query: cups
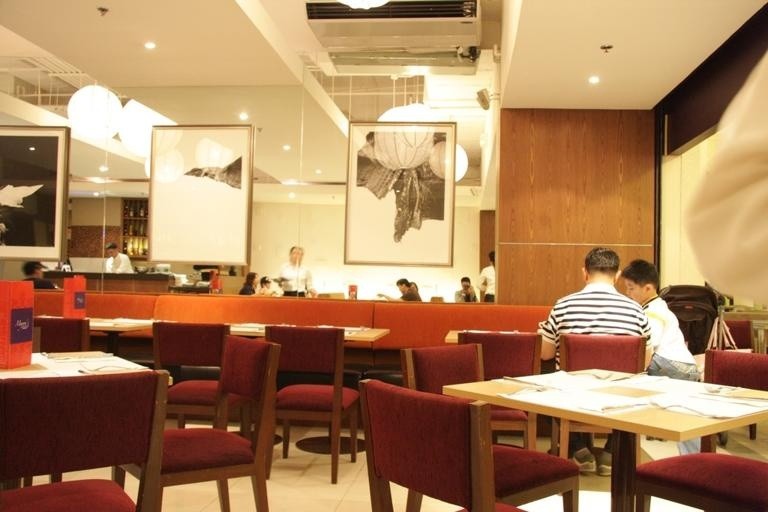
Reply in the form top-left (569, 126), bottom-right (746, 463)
top-left (349, 285), bottom-right (357, 300)
top-left (753, 297), bottom-right (763, 311)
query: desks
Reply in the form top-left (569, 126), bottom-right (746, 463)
top-left (445, 330), bottom-right (537, 345)
top-left (171, 286), bottom-right (210, 294)
top-left (442, 367), bottom-right (768, 512)
top-left (0, 351), bottom-right (174, 491)
top-left (227, 322), bottom-right (390, 342)
top-left (35, 315), bottom-right (176, 331)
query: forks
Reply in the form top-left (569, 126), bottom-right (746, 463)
top-left (717, 385), bottom-right (742, 391)
top-left (657, 403), bottom-right (705, 417)
top-left (85, 364), bottom-right (130, 372)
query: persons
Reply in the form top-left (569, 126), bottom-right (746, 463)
top-left (536, 246), bottom-right (655, 477)
top-left (454, 276), bottom-right (478, 302)
top-left (621, 259), bottom-right (702, 456)
top-left (21, 261), bottom-right (55, 289)
top-left (239, 272), bottom-right (259, 295)
top-left (408, 282), bottom-right (418, 291)
top-left (384, 278), bottom-right (423, 302)
top-left (259, 276), bottom-right (272, 296)
top-left (105, 242), bottom-right (134, 273)
top-left (272, 246), bottom-right (316, 297)
top-left (476, 250), bottom-right (496, 303)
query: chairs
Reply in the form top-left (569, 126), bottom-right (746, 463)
top-left (0, 369), bottom-right (169, 512)
top-left (633, 453), bottom-right (768, 511)
top-left (152, 322), bottom-right (252, 443)
top-left (33, 316), bottom-right (90, 353)
top-left (403, 343), bottom-right (526, 512)
top-left (457, 332), bottom-right (542, 451)
top-left (706, 350), bottom-right (768, 390)
top-left (266, 326), bottom-right (361, 484)
top-left (363, 378), bottom-right (579, 512)
top-left (110, 335), bottom-right (280, 512)
top-left (549, 334), bottom-right (650, 460)
top-left (724, 320), bottom-right (753, 351)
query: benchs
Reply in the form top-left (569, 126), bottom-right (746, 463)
top-left (352, 305), bottom-right (554, 385)
top-left (151, 295), bottom-right (374, 390)
top-left (32, 292), bottom-right (156, 367)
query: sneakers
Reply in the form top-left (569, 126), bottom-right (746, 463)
top-left (598, 464), bottom-right (612, 476)
top-left (571, 453), bottom-right (596, 473)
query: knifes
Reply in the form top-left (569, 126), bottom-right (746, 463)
top-left (602, 404), bottom-right (649, 412)
top-left (612, 372), bottom-right (648, 381)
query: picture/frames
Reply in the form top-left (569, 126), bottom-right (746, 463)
top-left (0, 125), bottom-right (70, 261)
top-left (347, 120), bottom-right (456, 268)
top-left (146, 124), bottom-right (253, 268)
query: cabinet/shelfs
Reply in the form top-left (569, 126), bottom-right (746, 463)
top-left (42, 271), bottom-right (176, 294)
top-left (123, 199), bottom-right (147, 258)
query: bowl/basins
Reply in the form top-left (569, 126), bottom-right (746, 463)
top-left (135, 266), bottom-right (149, 273)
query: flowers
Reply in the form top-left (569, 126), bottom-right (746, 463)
top-left (355, 129), bottom-right (447, 241)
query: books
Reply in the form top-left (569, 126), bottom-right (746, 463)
top-left (0, 279), bottom-right (35, 370)
top-left (62, 277), bottom-right (86, 319)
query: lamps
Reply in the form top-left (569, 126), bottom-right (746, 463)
top-left (396, 104), bottom-right (430, 127)
top-left (146, 41), bottom-right (154, 49)
top-left (67, 86), bottom-right (123, 141)
top-left (145, 149), bottom-right (152, 177)
top-left (454, 145), bottom-right (468, 183)
top-left (589, 75), bottom-right (596, 83)
top-left (119, 99), bottom-right (180, 156)
top-left (239, 112), bottom-right (247, 120)
top-left (339, 0), bottom-right (387, 9)
top-left (377, 105), bottom-right (397, 124)
